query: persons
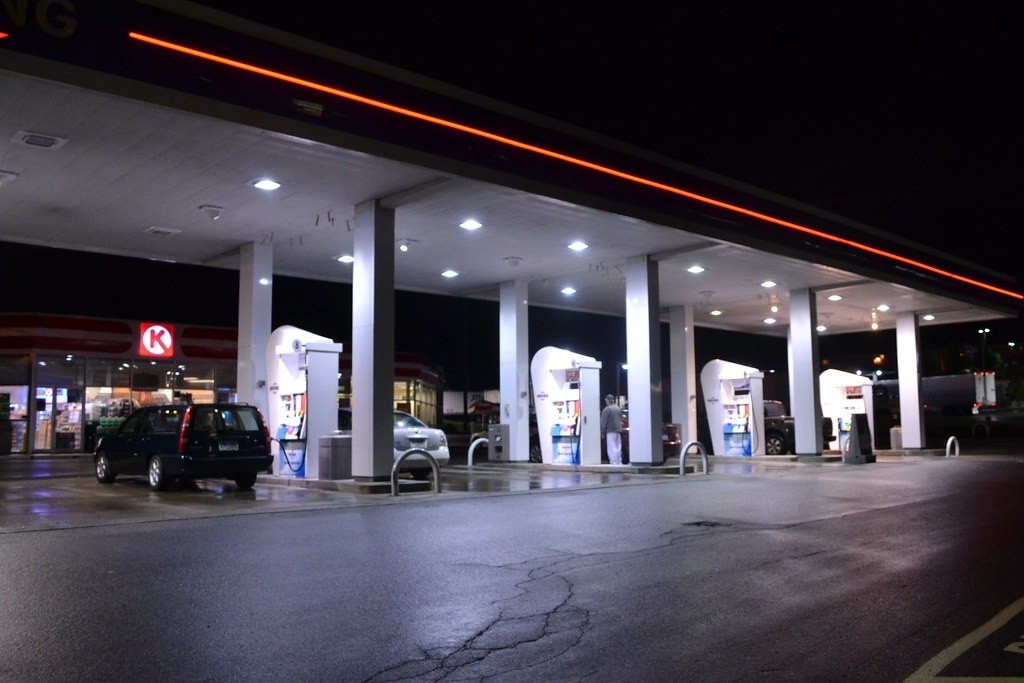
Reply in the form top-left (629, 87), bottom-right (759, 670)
top-left (601, 394), bottom-right (624, 466)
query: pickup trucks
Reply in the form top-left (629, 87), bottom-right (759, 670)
top-left (762, 400), bottom-right (837, 455)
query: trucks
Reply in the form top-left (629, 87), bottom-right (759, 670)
top-left (872, 371), bottom-right (1002, 449)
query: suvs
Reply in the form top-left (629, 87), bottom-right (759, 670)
top-left (94, 403), bottom-right (274, 490)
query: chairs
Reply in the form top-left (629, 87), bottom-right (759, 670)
top-left (150, 414), bottom-right (237, 432)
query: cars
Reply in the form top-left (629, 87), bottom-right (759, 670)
top-left (338, 407), bottom-right (449, 479)
top-left (601, 408), bottom-right (683, 465)
